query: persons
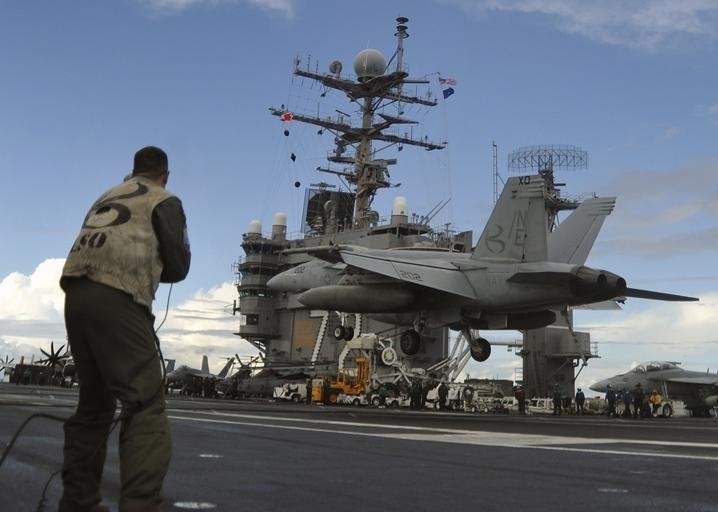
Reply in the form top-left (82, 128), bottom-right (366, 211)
top-left (462, 382), bottom-right (474, 405)
top-left (604, 382), bottom-right (661, 418)
top-left (306, 376), bottom-right (330, 405)
top-left (365, 380), bottom-right (387, 408)
top-left (515, 386), bottom-right (525, 412)
top-left (552, 384), bottom-right (561, 414)
top-left (575, 388), bottom-right (585, 416)
top-left (57, 146), bottom-right (192, 512)
top-left (410, 378), bottom-right (448, 411)
top-left (192, 376), bottom-right (216, 398)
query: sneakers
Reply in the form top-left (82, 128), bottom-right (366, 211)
top-left (159, 498), bottom-right (175, 510)
top-left (60, 502), bottom-right (109, 511)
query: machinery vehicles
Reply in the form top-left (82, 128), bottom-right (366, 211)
top-left (329, 358), bottom-right (370, 403)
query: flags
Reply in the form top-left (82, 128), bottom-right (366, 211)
top-left (438, 79), bottom-right (458, 86)
top-left (281, 112), bottom-right (293, 122)
top-left (442, 87), bottom-right (454, 98)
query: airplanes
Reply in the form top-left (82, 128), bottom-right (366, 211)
top-left (589, 363), bottom-right (718, 418)
top-left (266, 175), bottom-right (699, 362)
top-left (1, 344), bottom-right (258, 399)
